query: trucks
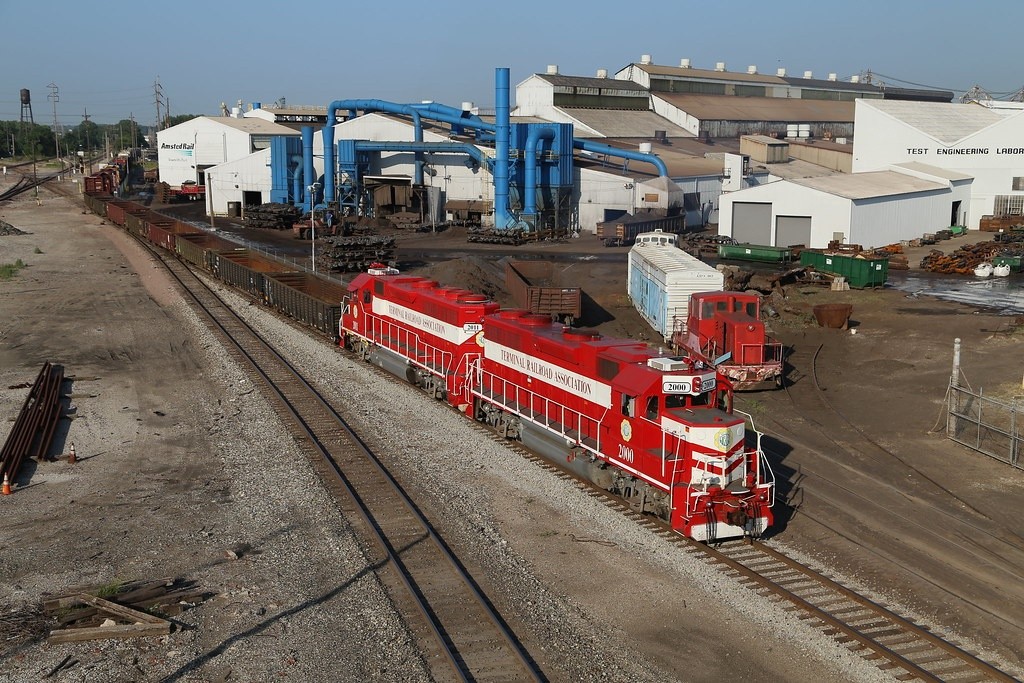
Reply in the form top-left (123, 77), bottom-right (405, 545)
top-left (627, 240), bottom-right (784, 394)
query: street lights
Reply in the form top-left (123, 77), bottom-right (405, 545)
top-left (306, 182), bottom-right (321, 273)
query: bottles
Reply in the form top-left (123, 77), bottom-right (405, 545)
top-left (70, 443), bottom-right (76, 462)
top-left (3, 472), bottom-right (10, 495)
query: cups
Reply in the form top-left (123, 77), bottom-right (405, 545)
top-left (851, 329), bottom-right (856, 334)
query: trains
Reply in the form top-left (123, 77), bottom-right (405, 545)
top-left (83, 147), bottom-right (777, 543)
